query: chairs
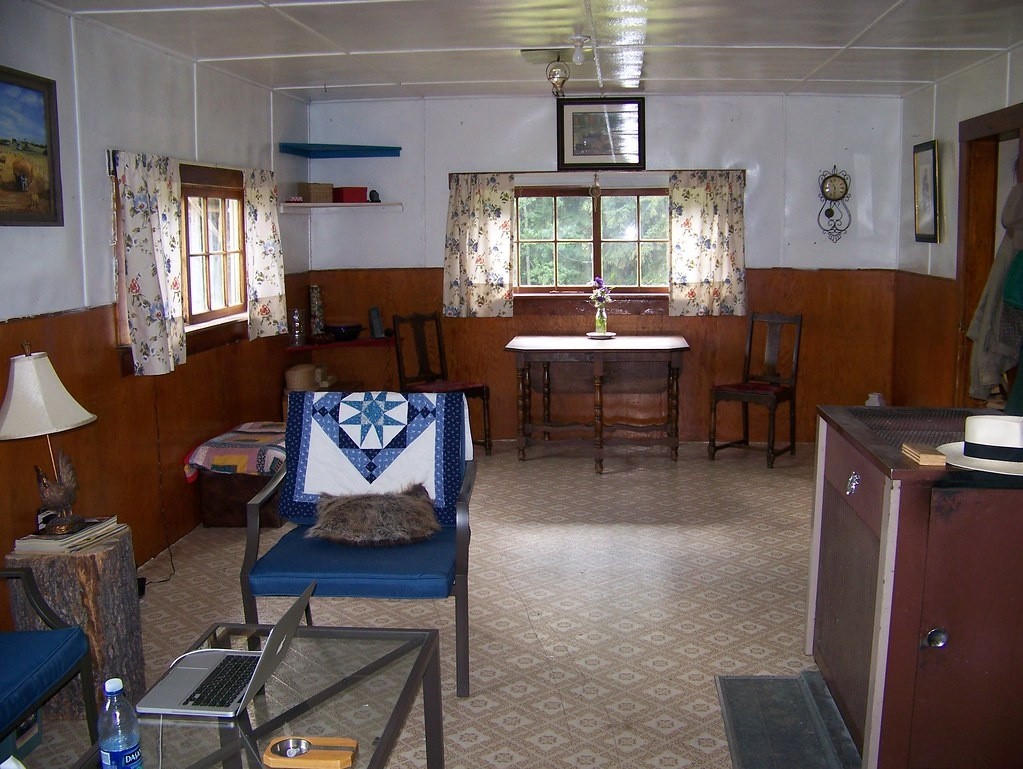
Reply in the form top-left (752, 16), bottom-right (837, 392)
top-left (393, 310), bottom-right (493, 456)
top-left (709, 311), bottom-right (804, 470)
top-left (239, 390), bottom-right (477, 699)
top-left (0, 567), bottom-right (99, 747)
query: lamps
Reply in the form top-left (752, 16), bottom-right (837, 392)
top-left (0, 339), bottom-right (98, 535)
top-left (545, 56), bottom-right (570, 97)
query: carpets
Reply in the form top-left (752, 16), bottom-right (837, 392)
top-left (713, 669), bottom-right (863, 769)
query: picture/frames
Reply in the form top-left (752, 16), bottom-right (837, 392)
top-left (368, 307), bottom-right (385, 339)
top-left (0, 64), bottom-right (64, 227)
top-left (913, 139), bottom-right (940, 244)
top-left (556, 98), bottom-right (646, 172)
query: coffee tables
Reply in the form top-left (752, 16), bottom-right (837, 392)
top-left (70, 622), bottom-right (445, 769)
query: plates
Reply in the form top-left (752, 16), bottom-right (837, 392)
top-left (586, 332), bottom-right (617, 338)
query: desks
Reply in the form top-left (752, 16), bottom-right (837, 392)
top-left (6, 523), bottom-right (147, 721)
top-left (504, 335), bottom-right (691, 475)
top-left (281, 381), bottom-right (364, 422)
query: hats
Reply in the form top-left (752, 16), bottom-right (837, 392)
top-left (935, 414), bottom-right (1023, 477)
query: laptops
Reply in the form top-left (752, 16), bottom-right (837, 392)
top-left (135, 580), bottom-right (318, 718)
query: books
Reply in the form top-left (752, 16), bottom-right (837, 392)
top-left (14, 514), bottom-right (127, 553)
top-left (902, 443), bottom-right (947, 466)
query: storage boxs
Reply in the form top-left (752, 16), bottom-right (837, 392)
top-left (333, 187), bottom-right (367, 202)
top-left (297, 182), bottom-right (333, 203)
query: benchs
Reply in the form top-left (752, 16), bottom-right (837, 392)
top-left (184, 422), bottom-right (288, 527)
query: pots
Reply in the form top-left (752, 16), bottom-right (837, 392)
top-left (320, 323), bottom-right (367, 340)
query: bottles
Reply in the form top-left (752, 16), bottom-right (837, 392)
top-left (96, 678), bottom-right (144, 769)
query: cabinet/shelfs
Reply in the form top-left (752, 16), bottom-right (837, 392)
top-left (801, 405), bottom-right (1023, 769)
top-left (278, 142), bottom-right (403, 360)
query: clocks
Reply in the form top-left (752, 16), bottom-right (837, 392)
top-left (817, 164), bottom-right (852, 244)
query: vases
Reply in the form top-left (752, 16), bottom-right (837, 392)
top-left (595, 308), bottom-right (607, 335)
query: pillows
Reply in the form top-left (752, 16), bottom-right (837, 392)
top-left (305, 482), bottom-right (442, 549)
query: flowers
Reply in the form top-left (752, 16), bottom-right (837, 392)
top-left (584, 276), bottom-right (617, 331)
